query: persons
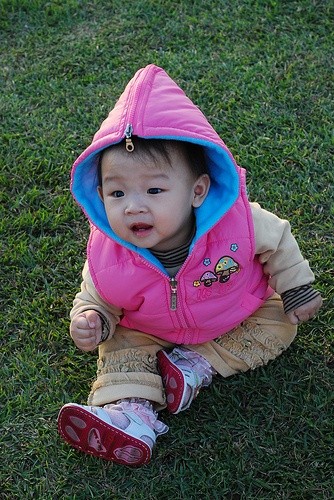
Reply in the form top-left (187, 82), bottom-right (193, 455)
top-left (55, 63), bottom-right (323, 468)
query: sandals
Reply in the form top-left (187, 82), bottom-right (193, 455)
top-left (156, 347), bottom-right (203, 414)
top-left (57, 401), bottom-right (157, 467)
